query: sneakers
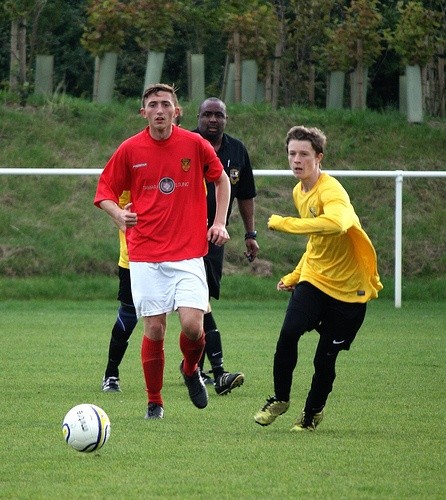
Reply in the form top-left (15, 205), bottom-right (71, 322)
top-left (213, 371), bottom-right (245, 396)
top-left (145, 401), bottom-right (165, 420)
top-left (253, 394), bottom-right (290, 426)
top-left (200, 370), bottom-right (214, 384)
top-left (101, 373), bottom-right (122, 392)
top-left (179, 359), bottom-right (209, 409)
top-left (289, 408), bottom-right (324, 435)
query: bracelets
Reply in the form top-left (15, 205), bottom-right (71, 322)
top-left (244, 231), bottom-right (257, 237)
top-left (245, 236), bottom-right (256, 241)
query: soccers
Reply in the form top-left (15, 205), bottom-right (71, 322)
top-left (62, 403), bottom-right (111, 453)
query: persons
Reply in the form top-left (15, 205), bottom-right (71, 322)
top-left (101, 97), bottom-right (260, 396)
top-left (253, 123), bottom-right (384, 433)
top-left (93, 83), bottom-right (232, 420)
